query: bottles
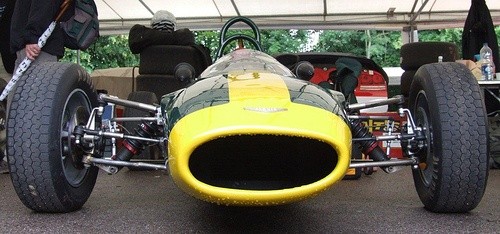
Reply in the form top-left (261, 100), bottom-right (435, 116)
top-left (437, 56), bottom-right (443, 63)
top-left (480, 43), bottom-right (493, 81)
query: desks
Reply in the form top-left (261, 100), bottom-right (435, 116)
top-left (477, 80), bottom-right (500, 101)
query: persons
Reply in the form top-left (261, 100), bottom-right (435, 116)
top-left (0, 0), bottom-right (74, 174)
top-left (151, 10), bottom-right (176, 32)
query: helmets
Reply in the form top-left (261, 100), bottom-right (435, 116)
top-left (151, 10), bottom-right (176, 32)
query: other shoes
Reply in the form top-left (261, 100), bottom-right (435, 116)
top-left (0, 161), bottom-right (10, 173)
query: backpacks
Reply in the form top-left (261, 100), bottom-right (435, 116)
top-left (60, 0), bottom-right (101, 50)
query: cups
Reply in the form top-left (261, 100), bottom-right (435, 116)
top-left (495, 73), bottom-right (500, 80)
